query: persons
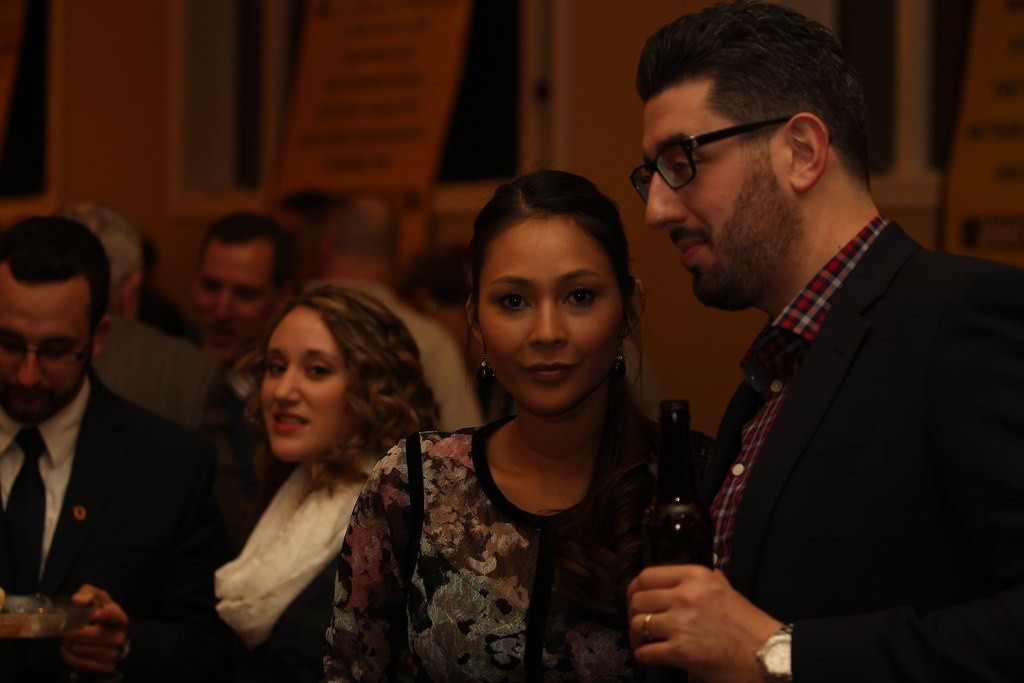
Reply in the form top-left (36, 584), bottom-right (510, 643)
top-left (626, 0), bottom-right (1024, 683)
top-left (216, 285), bottom-right (443, 683)
top-left (64, 200), bottom-right (243, 496)
top-left (304, 196), bottom-right (483, 433)
top-left (323, 170), bottom-right (714, 683)
top-left (198, 213), bottom-right (300, 502)
top-left (0, 212), bottom-right (233, 683)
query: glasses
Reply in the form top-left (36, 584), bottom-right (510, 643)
top-left (631, 113), bottom-right (839, 205)
top-left (0, 329), bottom-right (94, 369)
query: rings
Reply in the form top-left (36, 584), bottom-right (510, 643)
top-left (642, 615), bottom-right (654, 641)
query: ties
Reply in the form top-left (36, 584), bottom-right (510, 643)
top-left (4, 427), bottom-right (45, 594)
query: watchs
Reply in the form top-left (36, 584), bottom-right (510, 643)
top-left (756, 624), bottom-right (794, 683)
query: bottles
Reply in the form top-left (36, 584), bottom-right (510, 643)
top-left (647, 400), bottom-right (712, 568)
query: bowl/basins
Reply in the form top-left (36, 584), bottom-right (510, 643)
top-left (0, 595), bottom-right (98, 638)
